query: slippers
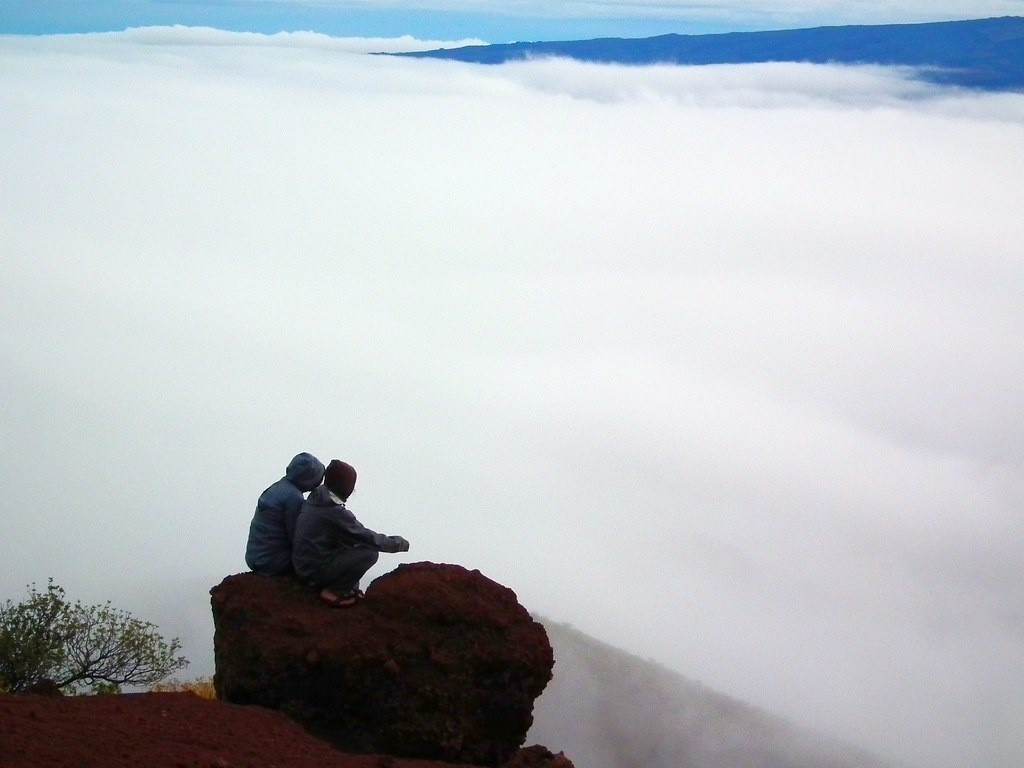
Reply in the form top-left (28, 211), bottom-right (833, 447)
top-left (327, 591), bottom-right (363, 608)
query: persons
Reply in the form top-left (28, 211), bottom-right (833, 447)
top-left (292, 459), bottom-right (409, 606)
top-left (245, 452), bottom-right (325, 575)
